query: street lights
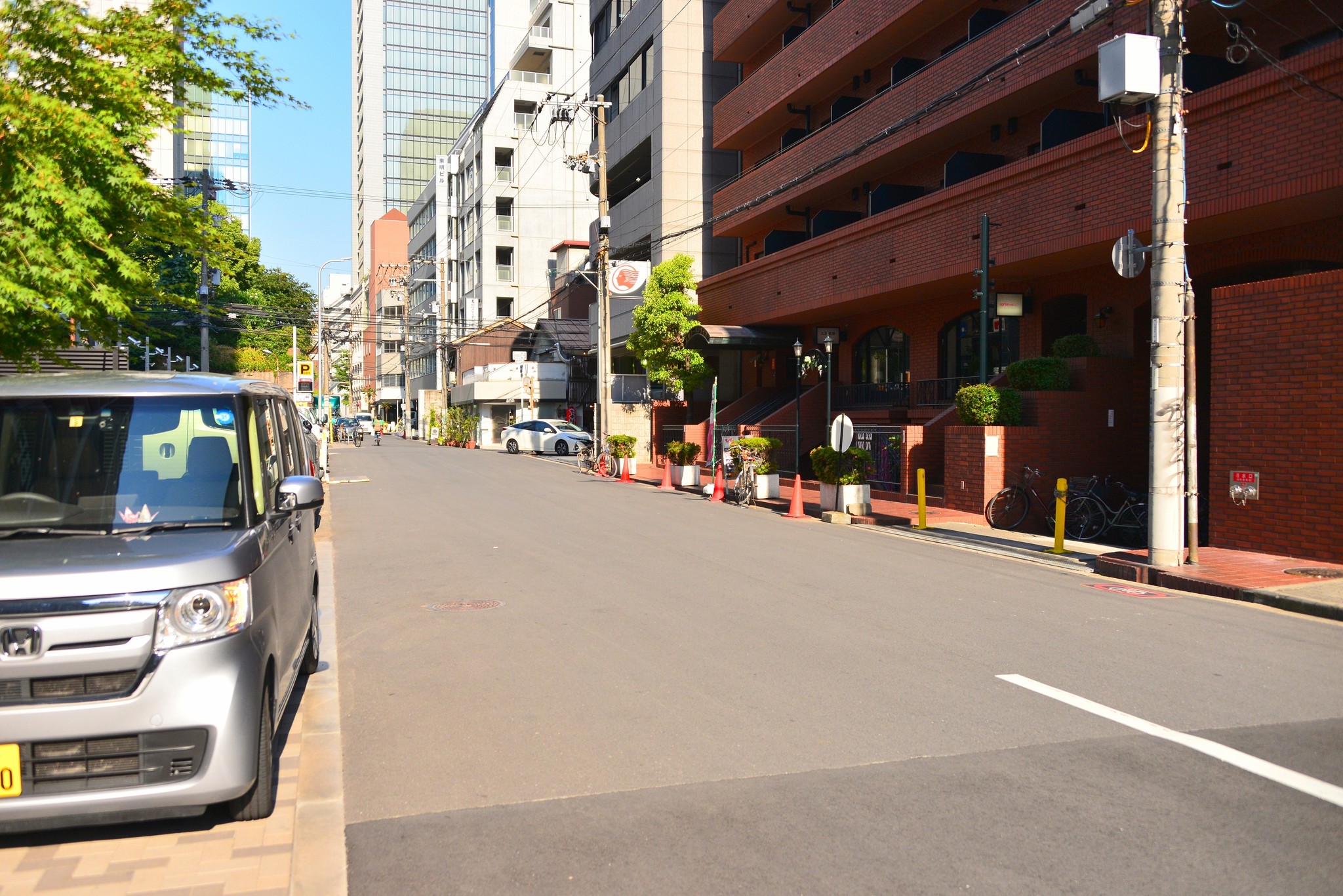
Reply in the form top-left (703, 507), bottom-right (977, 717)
top-left (317, 256), bottom-right (355, 427)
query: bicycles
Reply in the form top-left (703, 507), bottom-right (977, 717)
top-left (1064, 473), bottom-right (1150, 545)
top-left (724, 444), bottom-right (765, 508)
top-left (988, 463), bottom-right (1095, 541)
top-left (396, 421), bottom-right (403, 433)
top-left (350, 423), bottom-right (364, 448)
top-left (577, 432), bottom-right (618, 478)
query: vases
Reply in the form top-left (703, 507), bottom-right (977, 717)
top-left (612, 457), bottom-right (636, 475)
top-left (739, 473), bottom-right (781, 499)
top-left (820, 482), bottom-right (872, 515)
top-left (670, 464), bottom-right (700, 486)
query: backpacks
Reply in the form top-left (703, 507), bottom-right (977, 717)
top-left (374, 420), bottom-right (382, 431)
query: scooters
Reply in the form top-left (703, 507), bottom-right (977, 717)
top-left (372, 425), bottom-right (382, 446)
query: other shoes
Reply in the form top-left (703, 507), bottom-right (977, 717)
top-left (374, 439), bottom-right (377, 442)
top-left (379, 438), bottom-right (382, 439)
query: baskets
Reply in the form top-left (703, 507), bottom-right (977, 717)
top-left (601, 441), bottom-right (614, 453)
top-left (739, 452), bottom-right (763, 469)
top-left (1006, 468), bottom-right (1034, 489)
top-left (1068, 476), bottom-right (1091, 495)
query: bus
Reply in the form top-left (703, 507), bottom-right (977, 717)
top-left (402, 412), bottom-right (418, 429)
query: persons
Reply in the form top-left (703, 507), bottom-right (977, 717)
top-left (372, 415), bottom-right (384, 443)
top-left (331, 407), bottom-right (340, 418)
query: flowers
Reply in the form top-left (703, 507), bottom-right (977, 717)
top-left (666, 440), bottom-right (702, 466)
top-left (729, 436), bottom-right (784, 475)
top-left (605, 434), bottom-right (638, 459)
top-left (810, 445), bottom-right (877, 485)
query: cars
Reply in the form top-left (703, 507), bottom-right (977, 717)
top-left (295, 406), bottom-right (327, 482)
top-left (334, 418), bottom-right (364, 441)
top-left (501, 419), bottom-right (595, 454)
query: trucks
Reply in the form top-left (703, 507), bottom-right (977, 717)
top-left (313, 395), bottom-right (342, 424)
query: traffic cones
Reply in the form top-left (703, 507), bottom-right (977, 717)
top-left (706, 465), bottom-right (727, 503)
top-left (780, 474), bottom-right (812, 520)
top-left (657, 458), bottom-right (675, 490)
top-left (615, 454), bottom-right (635, 483)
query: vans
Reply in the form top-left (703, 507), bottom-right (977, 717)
top-left (0, 369), bottom-right (329, 834)
top-left (354, 413), bottom-right (373, 434)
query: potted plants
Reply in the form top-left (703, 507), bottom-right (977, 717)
top-left (437, 401), bottom-right (480, 449)
